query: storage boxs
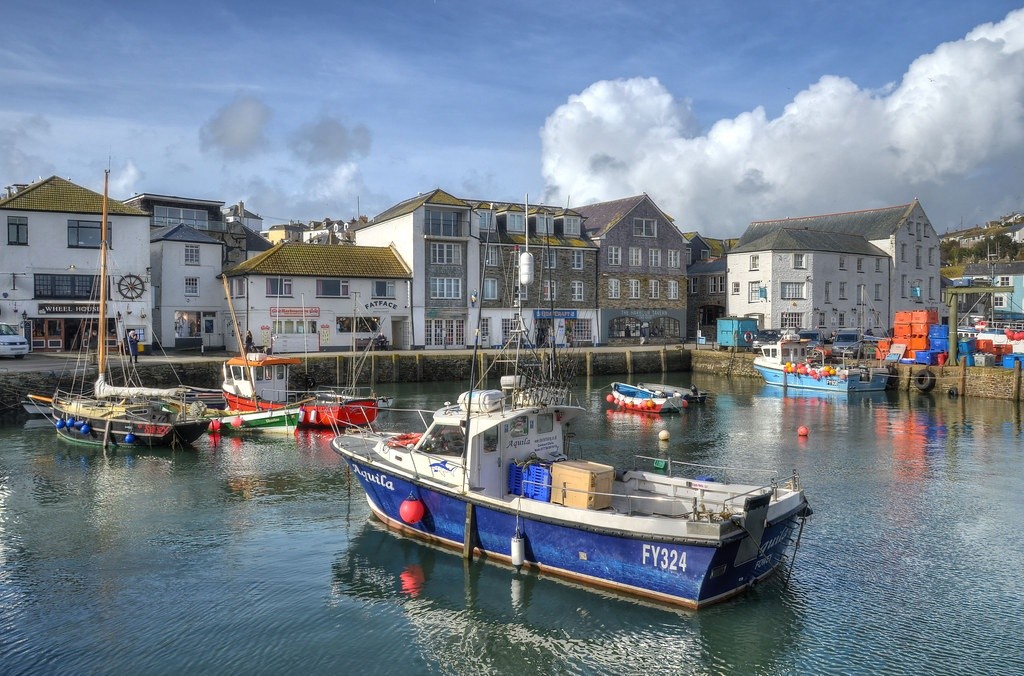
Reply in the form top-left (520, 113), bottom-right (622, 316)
top-left (506, 463), bottom-right (554, 501)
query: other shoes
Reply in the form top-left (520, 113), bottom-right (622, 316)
top-left (135, 362), bottom-right (139, 363)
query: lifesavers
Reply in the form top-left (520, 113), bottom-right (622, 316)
top-left (948, 387), bottom-right (958, 394)
top-left (913, 368), bottom-right (936, 391)
top-left (385, 432), bottom-right (422, 448)
top-left (743, 331), bottom-right (753, 342)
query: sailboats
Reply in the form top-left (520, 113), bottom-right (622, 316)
top-left (2, 156), bottom-right (315, 449)
top-left (221, 274), bottom-right (394, 429)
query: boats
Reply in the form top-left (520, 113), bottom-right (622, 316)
top-left (323, 193), bottom-right (814, 610)
top-left (753, 283), bottom-right (891, 393)
top-left (604, 382), bottom-right (712, 414)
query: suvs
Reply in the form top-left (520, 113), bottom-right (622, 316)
top-left (752, 329), bottom-right (784, 354)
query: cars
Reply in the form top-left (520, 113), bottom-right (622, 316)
top-left (0, 322), bottom-right (29, 358)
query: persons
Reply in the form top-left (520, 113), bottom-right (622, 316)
top-left (245, 330), bottom-right (252, 353)
top-left (129, 330), bottom-right (140, 364)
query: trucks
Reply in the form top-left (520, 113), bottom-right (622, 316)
top-left (831, 328), bottom-right (865, 358)
